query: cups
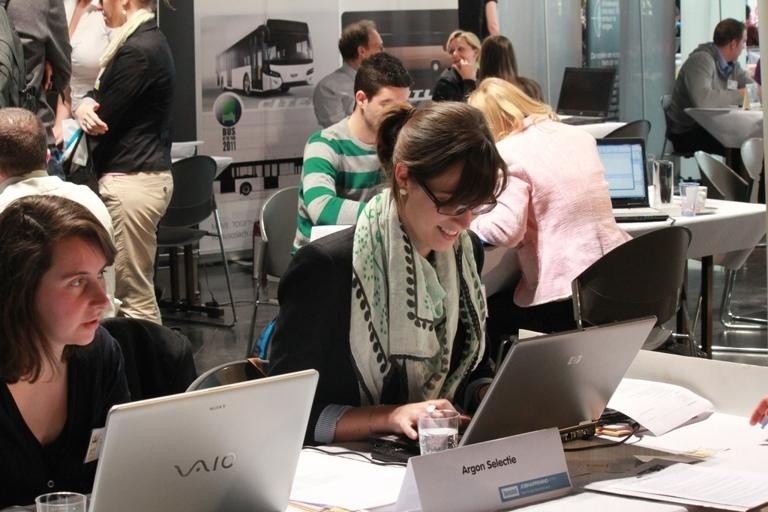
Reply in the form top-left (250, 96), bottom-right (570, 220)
top-left (679, 182), bottom-right (700, 217)
top-left (417, 410), bottom-right (459, 456)
top-left (35, 492), bottom-right (87, 512)
top-left (686, 186), bottom-right (708, 213)
top-left (653, 160), bottom-right (675, 204)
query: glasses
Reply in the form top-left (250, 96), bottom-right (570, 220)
top-left (417, 178), bottom-right (497, 216)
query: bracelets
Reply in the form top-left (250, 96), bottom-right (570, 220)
top-left (368, 404), bottom-right (387, 435)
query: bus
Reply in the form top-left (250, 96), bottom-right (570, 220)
top-left (213, 18), bottom-right (316, 98)
top-left (375, 28), bottom-right (457, 74)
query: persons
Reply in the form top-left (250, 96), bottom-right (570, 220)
top-left (429, 28), bottom-right (483, 102)
top-left (0, 193), bottom-right (133, 510)
top-left (667, 17), bottom-right (761, 156)
top-left (288, 51), bottom-right (416, 259)
top-left (746, 4), bottom-right (758, 47)
top-left (458, 0), bottom-right (499, 43)
top-left (312, 17), bottom-right (386, 128)
top-left (463, 71), bottom-right (633, 358)
top-left (477, 34), bottom-right (543, 104)
top-left (267, 98), bottom-right (511, 445)
top-left (1, 1), bottom-right (177, 327)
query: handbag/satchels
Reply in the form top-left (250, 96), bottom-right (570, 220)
top-left (62, 128), bottom-right (99, 195)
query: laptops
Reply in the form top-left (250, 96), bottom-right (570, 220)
top-left (596, 137), bottom-right (669, 223)
top-left (557, 68), bottom-right (616, 118)
top-left (369, 315), bottom-right (658, 462)
top-left (0, 369), bottom-right (320, 512)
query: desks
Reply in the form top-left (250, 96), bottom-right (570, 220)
top-left (0, 417), bottom-right (766, 511)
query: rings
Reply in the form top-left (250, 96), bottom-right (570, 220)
top-left (424, 404), bottom-right (437, 415)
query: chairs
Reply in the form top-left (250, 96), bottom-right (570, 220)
top-left (244, 187), bottom-right (299, 357)
top-left (151, 139), bottom-right (237, 331)
